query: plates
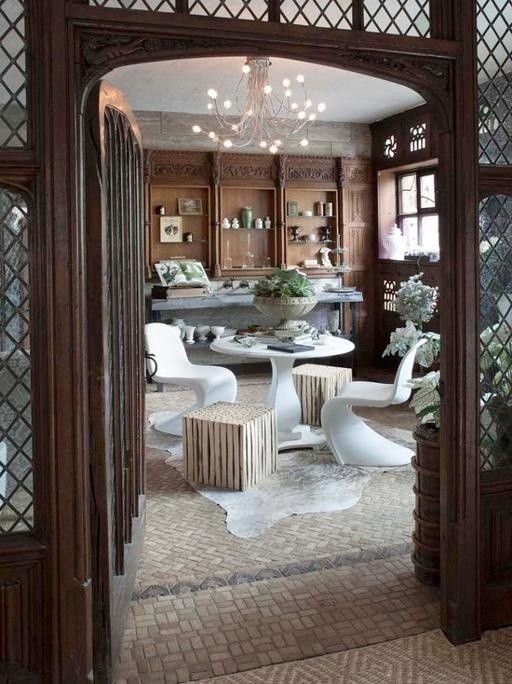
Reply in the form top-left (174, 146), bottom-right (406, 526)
top-left (238, 329), bottom-right (270, 335)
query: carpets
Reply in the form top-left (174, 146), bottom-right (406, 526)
top-left (147, 413), bottom-right (412, 539)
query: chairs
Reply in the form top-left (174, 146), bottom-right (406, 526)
top-left (320, 338), bottom-right (429, 466)
top-left (144, 322), bottom-right (238, 437)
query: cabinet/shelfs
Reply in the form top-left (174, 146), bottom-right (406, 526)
top-left (143, 151), bottom-right (341, 281)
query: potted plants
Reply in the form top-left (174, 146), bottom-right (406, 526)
top-left (381, 271), bottom-right (512, 586)
top-left (251, 268), bottom-right (318, 341)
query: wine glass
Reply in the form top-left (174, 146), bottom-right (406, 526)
top-left (210, 326), bottom-right (226, 341)
top-left (183, 326), bottom-right (196, 343)
top-left (195, 324), bottom-right (210, 341)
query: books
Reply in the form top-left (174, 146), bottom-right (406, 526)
top-left (267, 341), bottom-right (316, 353)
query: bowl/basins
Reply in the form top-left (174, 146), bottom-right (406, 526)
top-left (248, 325), bottom-right (263, 332)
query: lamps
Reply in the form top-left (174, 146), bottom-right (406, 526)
top-left (191, 56), bottom-right (328, 154)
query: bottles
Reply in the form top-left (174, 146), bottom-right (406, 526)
top-left (223, 240), bottom-right (233, 270)
top-left (259, 237), bottom-right (272, 269)
top-left (241, 230), bottom-right (256, 269)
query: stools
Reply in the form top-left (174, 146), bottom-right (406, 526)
top-left (181, 401), bottom-right (277, 491)
top-left (292, 363), bottom-right (353, 427)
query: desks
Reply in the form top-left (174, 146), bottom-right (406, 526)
top-left (209, 334), bottom-right (355, 452)
